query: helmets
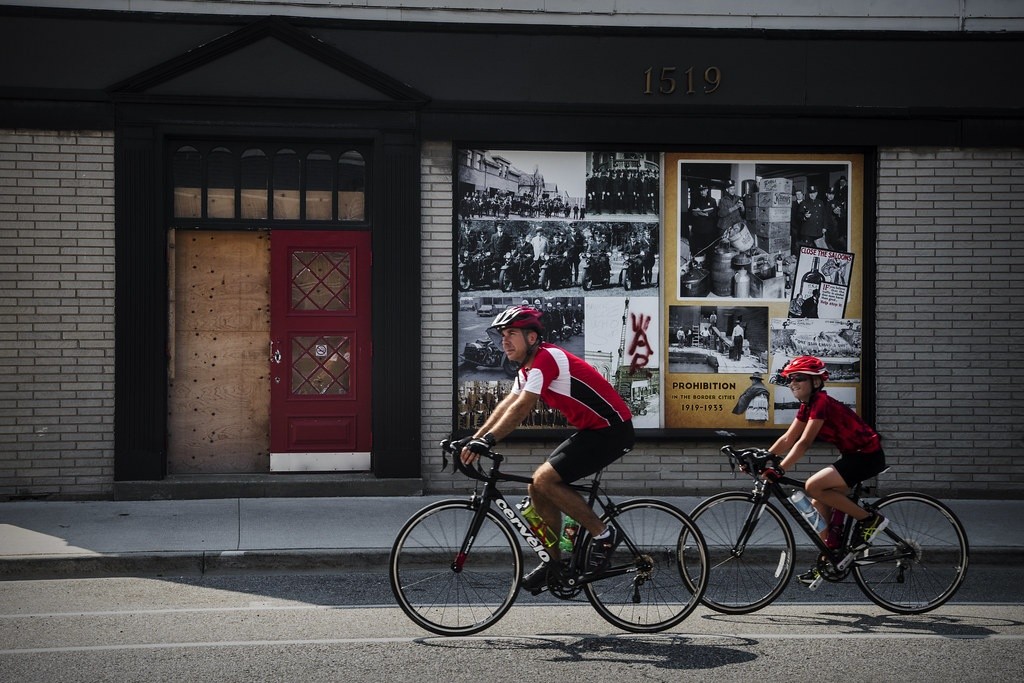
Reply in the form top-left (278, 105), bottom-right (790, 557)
top-left (780, 356), bottom-right (829, 381)
top-left (485, 306), bottom-right (546, 335)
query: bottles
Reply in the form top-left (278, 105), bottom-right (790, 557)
top-left (559, 514), bottom-right (579, 553)
top-left (790, 488), bottom-right (827, 533)
top-left (515, 496), bottom-right (558, 548)
top-left (777, 251), bottom-right (783, 272)
top-left (761, 261), bottom-right (775, 278)
top-left (825, 507), bottom-right (845, 548)
top-left (784, 276), bottom-right (792, 299)
top-left (800, 256), bottom-right (825, 301)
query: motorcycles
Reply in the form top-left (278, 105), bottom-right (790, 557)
top-left (459, 322), bottom-right (582, 378)
top-left (540, 255), bottom-right (573, 291)
top-left (457, 250), bottom-right (541, 292)
top-left (579, 253), bottom-right (610, 291)
top-left (622, 251), bottom-right (645, 290)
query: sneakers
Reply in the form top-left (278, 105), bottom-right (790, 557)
top-left (849, 509), bottom-right (890, 550)
top-left (796, 565), bottom-right (820, 584)
top-left (521, 561), bottom-right (551, 591)
top-left (581, 524), bottom-right (626, 580)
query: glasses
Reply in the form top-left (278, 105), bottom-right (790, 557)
top-left (786, 376), bottom-right (807, 382)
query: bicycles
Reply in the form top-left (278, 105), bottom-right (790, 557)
top-left (677, 445), bottom-right (969, 615)
top-left (389, 436), bottom-right (710, 636)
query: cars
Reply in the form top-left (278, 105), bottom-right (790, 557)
top-left (477, 305), bottom-right (498, 317)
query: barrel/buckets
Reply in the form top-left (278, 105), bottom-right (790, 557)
top-left (723, 222), bottom-right (754, 252)
top-left (708, 248), bottom-right (739, 297)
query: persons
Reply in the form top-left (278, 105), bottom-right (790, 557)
top-left (744, 356), bottom-right (888, 587)
top-left (458, 167), bottom-right (859, 427)
top-left (454, 305), bottom-right (635, 589)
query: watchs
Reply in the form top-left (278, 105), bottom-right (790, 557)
top-left (482, 432), bottom-right (498, 447)
